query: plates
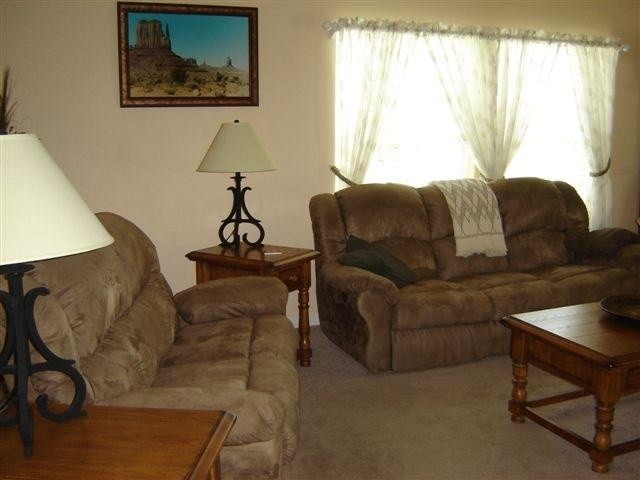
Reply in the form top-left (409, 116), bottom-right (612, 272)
top-left (600, 292), bottom-right (640, 321)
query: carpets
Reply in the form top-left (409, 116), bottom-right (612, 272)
top-left (294, 325), bottom-right (640, 479)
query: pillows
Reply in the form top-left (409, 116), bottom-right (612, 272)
top-left (336, 234), bottom-right (419, 289)
top-left (570, 227), bottom-right (636, 261)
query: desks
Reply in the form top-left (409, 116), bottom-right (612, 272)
top-left (0, 401), bottom-right (237, 478)
top-left (185, 241), bottom-right (322, 367)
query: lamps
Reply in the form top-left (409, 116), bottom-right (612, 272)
top-left (196, 119), bottom-right (282, 251)
top-left (0, 134), bottom-right (114, 458)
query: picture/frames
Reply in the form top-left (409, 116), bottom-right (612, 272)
top-left (116, 2), bottom-right (259, 108)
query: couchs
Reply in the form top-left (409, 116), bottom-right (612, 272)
top-left (309, 178), bottom-right (639, 375)
top-left (0, 211), bottom-right (301, 480)
